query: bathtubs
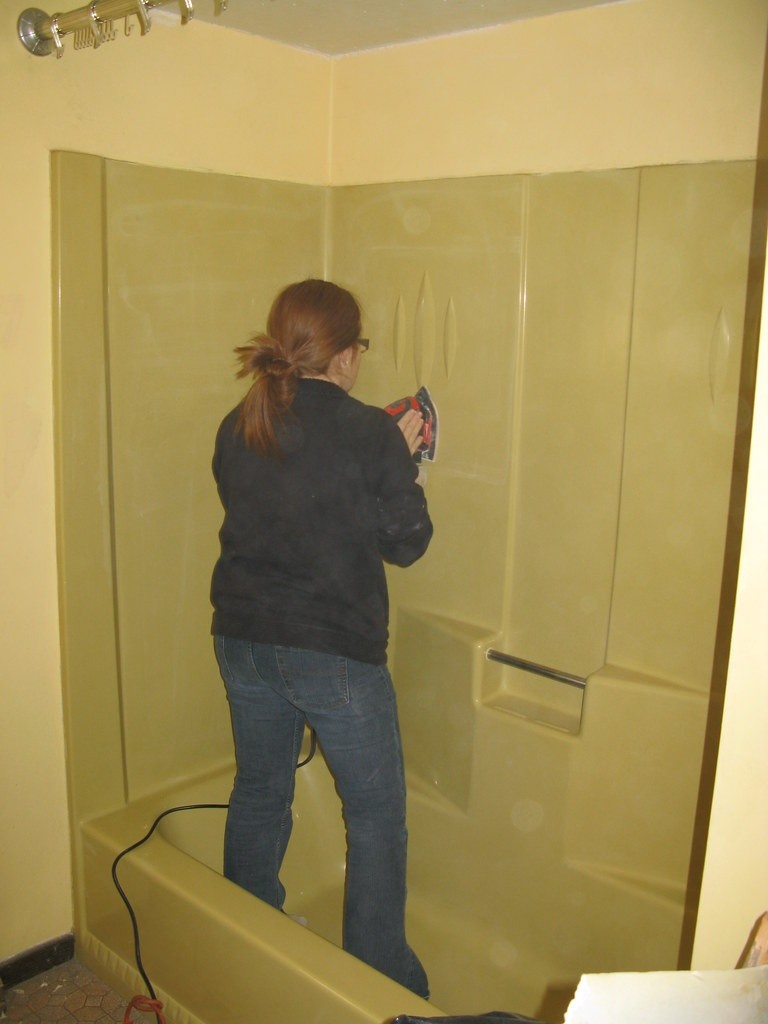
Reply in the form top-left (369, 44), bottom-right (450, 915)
top-left (77, 736), bottom-right (692, 1024)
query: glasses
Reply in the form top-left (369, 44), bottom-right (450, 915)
top-left (355, 339), bottom-right (369, 353)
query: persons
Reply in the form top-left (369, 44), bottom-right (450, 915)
top-left (209, 280), bottom-right (433, 1002)
